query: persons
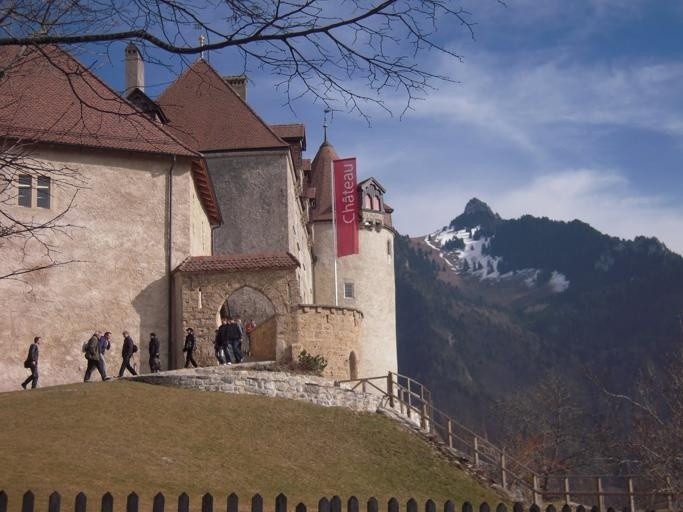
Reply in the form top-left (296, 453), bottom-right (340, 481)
top-left (182, 328), bottom-right (198, 368)
top-left (21, 337), bottom-right (41, 390)
top-left (91, 331), bottom-right (112, 377)
top-left (84, 331), bottom-right (111, 382)
top-left (214, 315), bottom-right (256, 367)
top-left (148, 332), bottom-right (160, 373)
top-left (113, 331), bottom-right (137, 377)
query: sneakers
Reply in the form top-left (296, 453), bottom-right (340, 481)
top-left (103, 377), bottom-right (110, 381)
top-left (84, 380), bottom-right (91, 382)
top-left (22, 383), bottom-right (25, 389)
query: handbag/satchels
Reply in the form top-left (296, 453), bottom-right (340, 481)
top-left (82, 340), bottom-right (89, 352)
top-left (152, 357), bottom-right (160, 369)
top-left (24, 360), bottom-right (29, 367)
top-left (133, 345), bottom-right (137, 352)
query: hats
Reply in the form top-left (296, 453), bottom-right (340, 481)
top-left (186, 328), bottom-right (193, 332)
